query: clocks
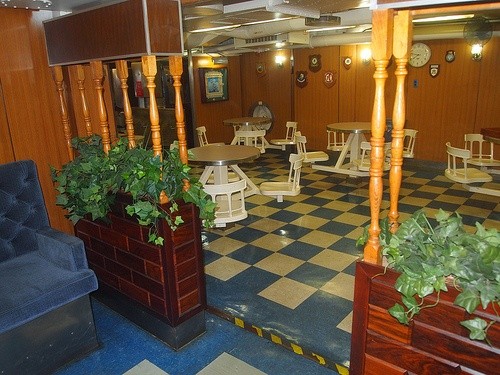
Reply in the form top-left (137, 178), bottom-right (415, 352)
top-left (408, 43), bottom-right (431, 67)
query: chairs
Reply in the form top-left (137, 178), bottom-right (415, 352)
top-left (271, 121), bottom-right (297, 150)
top-left (462, 133), bottom-right (500, 168)
top-left (352, 142), bottom-right (391, 171)
top-left (259, 153), bottom-right (304, 203)
top-left (195, 125), bottom-right (224, 147)
top-left (202, 179), bottom-right (248, 227)
top-left (294, 131), bottom-right (329, 163)
top-left (403, 129), bottom-right (419, 158)
top-left (0, 160), bottom-right (104, 375)
top-left (327, 130), bottom-right (347, 152)
top-left (235, 130), bottom-right (266, 153)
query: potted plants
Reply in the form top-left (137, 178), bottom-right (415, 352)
top-left (355, 208), bottom-right (500, 348)
top-left (48, 130), bottom-right (219, 246)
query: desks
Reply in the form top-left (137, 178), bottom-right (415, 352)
top-left (480, 128), bottom-right (500, 145)
top-left (326, 122), bottom-right (387, 178)
top-left (188, 145), bottom-right (262, 198)
top-left (223, 117), bottom-right (272, 152)
top-left (444, 142), bottom-right (493, 186)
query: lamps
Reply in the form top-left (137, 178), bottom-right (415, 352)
top-left (305, 16), bottom-right (341, 26)
